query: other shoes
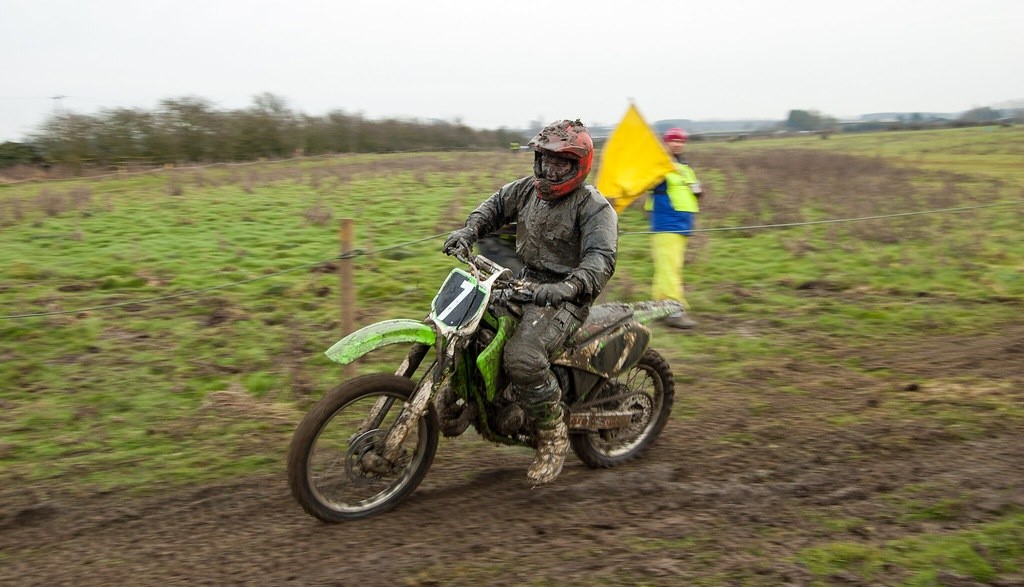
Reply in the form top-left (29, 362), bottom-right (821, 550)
top-left (663, 317), bottom-right (697, 329)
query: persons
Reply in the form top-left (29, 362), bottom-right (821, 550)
top-left (442, 120), bottom-right (618, 485)
top-left (645, 127), bottom-right (705, 327)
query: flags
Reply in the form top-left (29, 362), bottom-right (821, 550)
top-left (593, 105), bottom-right (677, 216)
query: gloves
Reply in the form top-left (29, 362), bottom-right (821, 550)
top-left (532, 280), bottom-right (578, 311)
top-left (441, 221), bottom-right (480, 256)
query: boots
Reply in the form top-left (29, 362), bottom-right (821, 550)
top-left (527, 410), bottom-right (571, 483)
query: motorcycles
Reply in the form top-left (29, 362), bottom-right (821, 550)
top-left (288, 241), bottom-right (676, 523)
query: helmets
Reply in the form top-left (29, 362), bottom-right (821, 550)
top-left (663, 127), bottom-right (688, 142)
top-left (527, 120), bottom-right (594, 201)
